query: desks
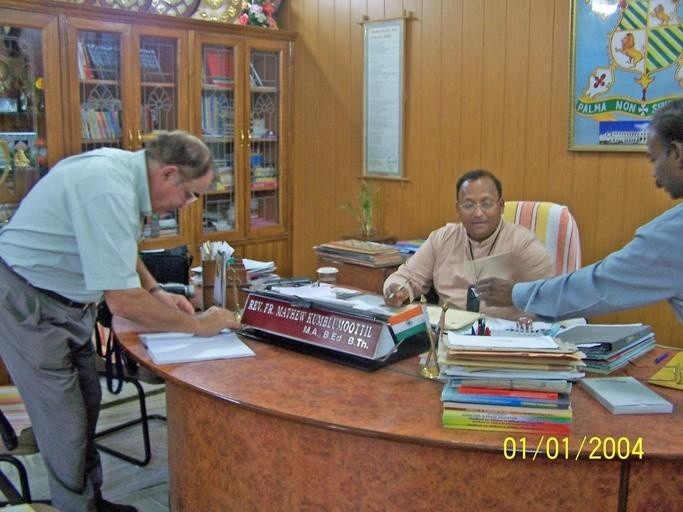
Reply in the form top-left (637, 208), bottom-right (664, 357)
top-left (343, 233), bottom-right (400, 247)
top-left (112, 269), bottom-right (682, 512)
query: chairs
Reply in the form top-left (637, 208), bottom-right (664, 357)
top-left (1, 396), bottom-right (100, 512)
top-left (499, 198), bottom-right (589, 331)
top-left (91, 244), bottom-right (199, 468)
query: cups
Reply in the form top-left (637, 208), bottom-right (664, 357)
top-left (315, 266), bottom-right (340, 286)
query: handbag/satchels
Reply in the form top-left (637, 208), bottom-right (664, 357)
top-left (97, 245), bottom-right (195, 330)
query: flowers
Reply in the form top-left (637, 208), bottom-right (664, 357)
top-left (238, 1), bottom-right (280, 29)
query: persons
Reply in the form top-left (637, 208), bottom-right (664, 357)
top-left (471, 94), bottom-right (683, 323)
top-left (381, 168), bottom-right (556, 323)
top-left (0, 127), bottom-right (242, 511)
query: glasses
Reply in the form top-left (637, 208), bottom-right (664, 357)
top-left (458, 198), bottom-right (500, 213)
top-left (179, 174), bottom-right (199, 205)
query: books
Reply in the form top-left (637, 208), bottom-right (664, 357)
top-left (136, 326), bottom-right (257, 364)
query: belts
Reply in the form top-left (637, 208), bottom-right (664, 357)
top-left (1, 256), bottom-right (94, 310)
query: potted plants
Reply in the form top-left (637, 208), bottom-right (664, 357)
top-left (339, 178), bottom-right (379, 237)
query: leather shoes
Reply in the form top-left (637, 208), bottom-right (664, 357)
top-left (97, 497), bottom-right (138, 512)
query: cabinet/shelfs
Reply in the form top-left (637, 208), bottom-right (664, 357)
top-left (1, 1), bottom-right (293, 316)
top-left (315, 251), bottom-right (436, 301)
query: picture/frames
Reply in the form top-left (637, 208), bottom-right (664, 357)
top-left (355, 10), bottom-right (417, 184)
top-left (565, 0), bottom-right (683, 154)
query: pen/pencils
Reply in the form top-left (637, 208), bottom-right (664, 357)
top-left (655, 349), bottom-right (672, 364)
top-left (471, 318), bottom-right (492, 336)
top-left (388, 277), bottom-right (412, 300)
top-left (419, 294), bottom-right (449, 376)
